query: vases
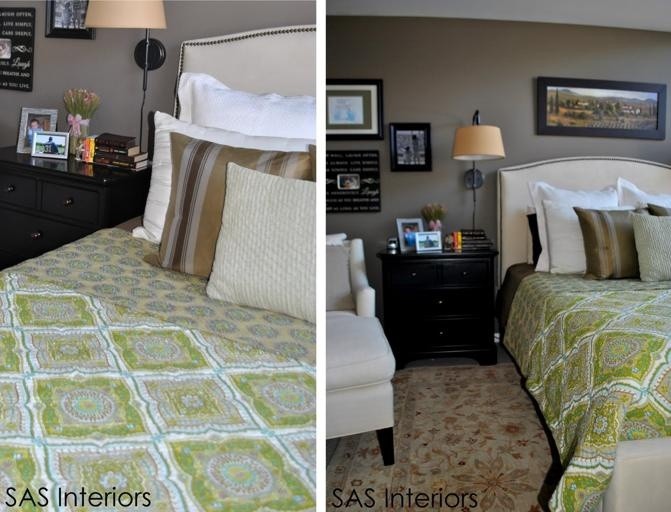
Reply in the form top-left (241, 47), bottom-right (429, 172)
top-left (428, 220), bottom-right (442, 231)
top-left (70, 119), bottom-right (90, 155)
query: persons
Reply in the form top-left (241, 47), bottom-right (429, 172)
top-left (26, 119), bottom-right (44, 145)
top-left (403, 146), bottom-right (412, 164)
top-left (340, 177), bottom-right (354, 188)
top-left (71, 0), bottom-right (81, 28)
top-left (43, 137), bottom-right (58, 154)
top-left (412, 134), bottom-right (422, 164)
top-left (404, 226), bottom-right (415, 246)
top-left (61, 1), bottom-right (73, 28)
top-left (424, 235), bottom-right (433, 247)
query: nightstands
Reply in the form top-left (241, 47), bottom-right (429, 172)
top-left (0, 145), bottom-right (151, 270)
top-left (376, 249), bottom-right (499, 370)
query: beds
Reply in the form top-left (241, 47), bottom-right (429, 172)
top-left (0, 24), bottom-right (316, 512)
top-left (496, 156), bottom-right (671, 512)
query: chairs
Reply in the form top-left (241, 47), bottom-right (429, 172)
top-left (326, 238), bottom-right (396, 466)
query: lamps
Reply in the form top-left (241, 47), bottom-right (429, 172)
top-left (85, 0), bottom-right (167, 152)
top-left (452, 109), bottom-right (506, 229)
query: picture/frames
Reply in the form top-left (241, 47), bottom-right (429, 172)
top-left (0, 7), bottom-right (35, 92)
top-left (389, 122), bottom-right (433, 172)
top-left (326, 77), bottom-right (384, 141)
top-left (415, 231), bottom-right (443, 251)
top-left (396, 217), bottom-right (423, 253)
top-left (45, 0), bottom-right (96, 40)
top-left (17, 106), bottom-right (58, 154)
top-left (326, 150), bottom-right (381, 214)
top-left (537, 76), bottom-right (666, 140)
top-left (31, 130), bottom-right (69, 160)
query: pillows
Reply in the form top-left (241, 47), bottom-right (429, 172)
top-left (144, 132), bottom-right (313, 278)
top-left (177, 71), bottom-right (316, 141)
top-left (205, 162), bottom-right (316, 327)
top-left (327, 245), bottom-right (356, 311)
top-left (326, 233), bottom-right (347, 246)
top-left (132, 110), bottom-right (316, 246)
top-left (309, 144), bottom-right (316, 181)
top-left (526, 176), bottom-right (671, 282)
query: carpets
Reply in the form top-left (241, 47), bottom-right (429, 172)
top-left (327, 341), bottom-right (562, 512)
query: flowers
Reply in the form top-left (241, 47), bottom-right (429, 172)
top-left (421, 202), bottom-right (448, 224)
top-left (63, 87), bottom-right (101, 119)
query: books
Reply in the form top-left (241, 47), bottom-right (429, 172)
top-left (81, 132), bottom-right (148, 169)
top-left (444, 228), bottom-right (493, 251)
